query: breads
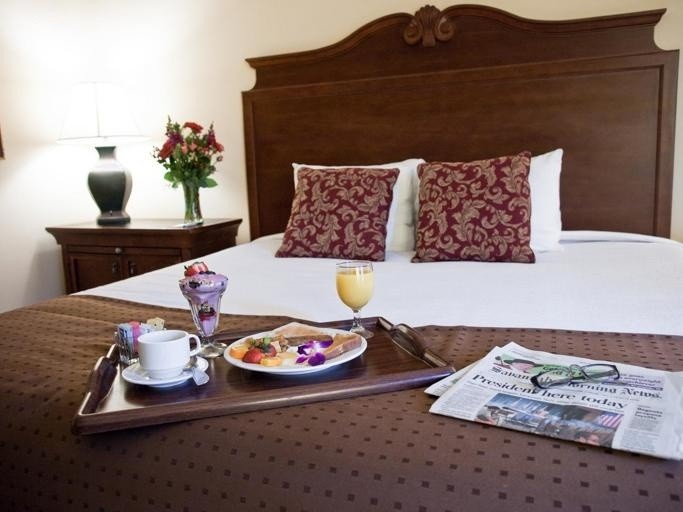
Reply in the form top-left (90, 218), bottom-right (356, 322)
top-left (322, 333), bottom-right (361, 361)
top-left (277, 326), bottom-right (333, 346)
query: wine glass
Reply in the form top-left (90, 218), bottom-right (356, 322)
top-left (179, 279), bottom-right (228, 358)
top-left (336, 260), bottom-right (375, 340)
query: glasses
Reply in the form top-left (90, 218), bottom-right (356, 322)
top-left (531, 364), bottom-right (621, 391)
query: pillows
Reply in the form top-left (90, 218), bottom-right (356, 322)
top-left (274, 148), bottom-right (565, 261)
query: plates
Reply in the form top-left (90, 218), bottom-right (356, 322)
top-left (120, 355), bottom-right (209, 388)
top-left (222, 326), bottom-right (367, 376)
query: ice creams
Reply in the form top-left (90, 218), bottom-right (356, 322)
top-left (178, 261), bottom-right (228, 337)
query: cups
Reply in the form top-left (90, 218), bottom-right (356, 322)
top-left (136, 330), bottom-right (201, 379)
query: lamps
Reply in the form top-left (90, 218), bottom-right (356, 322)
top-left (57, 87), bottom-right (148, 226)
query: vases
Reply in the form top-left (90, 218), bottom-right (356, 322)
top-left (179, 180), bottom-right (202, 226)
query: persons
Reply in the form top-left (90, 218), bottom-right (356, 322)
top-left (474, 403), bottom-right (624, 450)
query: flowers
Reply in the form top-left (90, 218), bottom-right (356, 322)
top-left (150, 117), bottom-right (224, 215)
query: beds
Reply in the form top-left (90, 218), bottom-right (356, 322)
top-left (0, 5), bottom-right (681, 510)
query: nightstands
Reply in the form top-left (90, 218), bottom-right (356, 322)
top-left (45, 216), bottom-right (241, 296)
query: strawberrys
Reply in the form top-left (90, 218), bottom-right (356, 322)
top-left (243, 344), bottom-right (277, 364)
